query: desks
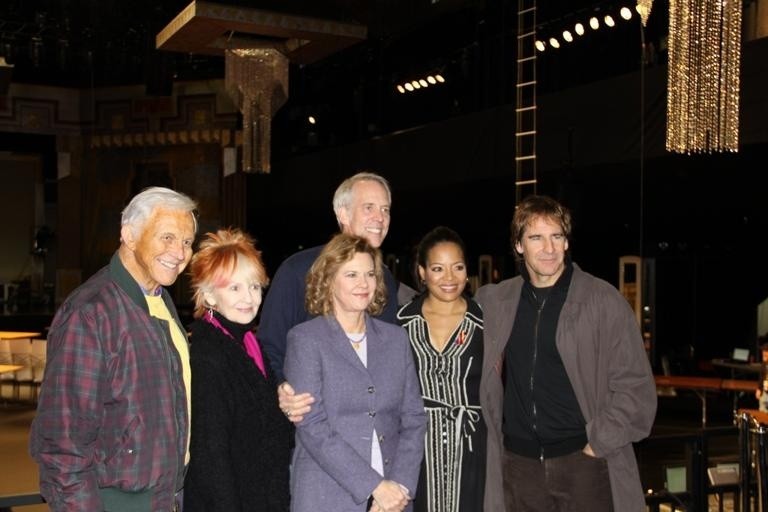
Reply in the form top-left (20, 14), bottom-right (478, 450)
top-left (651, 371), bottom-right (721, 424)
top-left (721, 377), bottom-right (764, 422)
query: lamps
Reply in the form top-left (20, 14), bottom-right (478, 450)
top-left (667, 0), bottom-right (744, 158)
top-left (219, 29), bottom-right (291, 178)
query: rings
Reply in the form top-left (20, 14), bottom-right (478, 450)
top-left (283, 410), bottom-right (291, 417)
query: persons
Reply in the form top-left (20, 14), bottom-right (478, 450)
top-left (280, 234), bottom-right (425, 512)
top-left (258, 171), bottom-right (405, 430)
top-left (390, 228), bottom-right (486, 511)
top-left (30, 160), bottom-right (197, 512)
top-left (475, 194), bottom-right (656, 511)
top-left (187, 230), bottom-right (290, 512)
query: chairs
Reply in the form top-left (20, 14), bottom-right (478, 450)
top-left (9, 336), bottom-right (36, 403)
top-left (29, 336), bottom-right (50, 402)
top-left (637, 430), bottom-right (698, 512)
top-left (698, 425), bottom-right (742, 512)
top-left (0, 336), bottom-right (18, 402)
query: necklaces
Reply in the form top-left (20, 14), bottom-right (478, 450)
top-left (344, 333), bottom-right (366, 350)
